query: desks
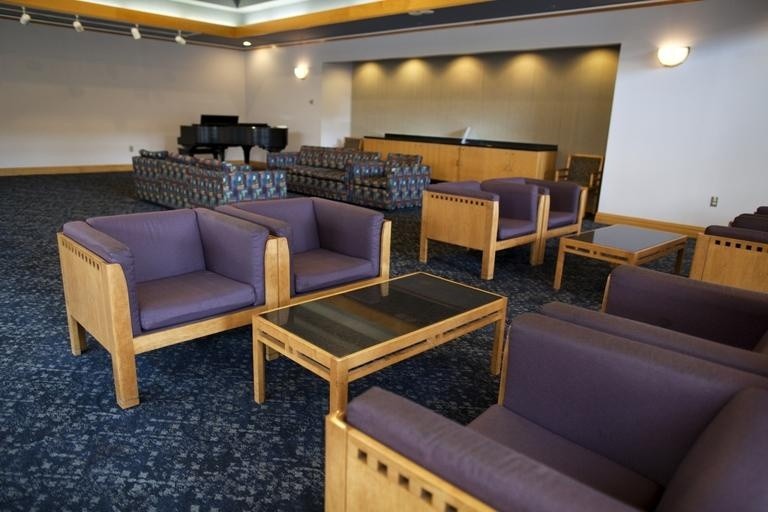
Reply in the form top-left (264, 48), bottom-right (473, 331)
top-left (252, 269), bottom-right (508, 414)
top-left (364, 132), bottom-right (558, 182)
top-left (553, 223), bottom-right (689, 290)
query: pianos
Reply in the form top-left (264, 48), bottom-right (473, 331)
top-left (175, 117), bottom-right (287, 161)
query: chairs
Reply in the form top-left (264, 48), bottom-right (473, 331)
top-left (540, 263), bottom-right (768, 375)
top-left (324, 312), bottom-right (767, 512)
top-left (554, 153), bottom-right (604, 214)
top-left (57, 206), bottom-right (279, 411)
top-left (482, 176), bottom-right (588, 265)
top-left (689, 206), bottom-right (767, 296)
top-left (215, 195), bottom-right (392, 358)
top-left (418, 180), bottom-right (545, 281)
